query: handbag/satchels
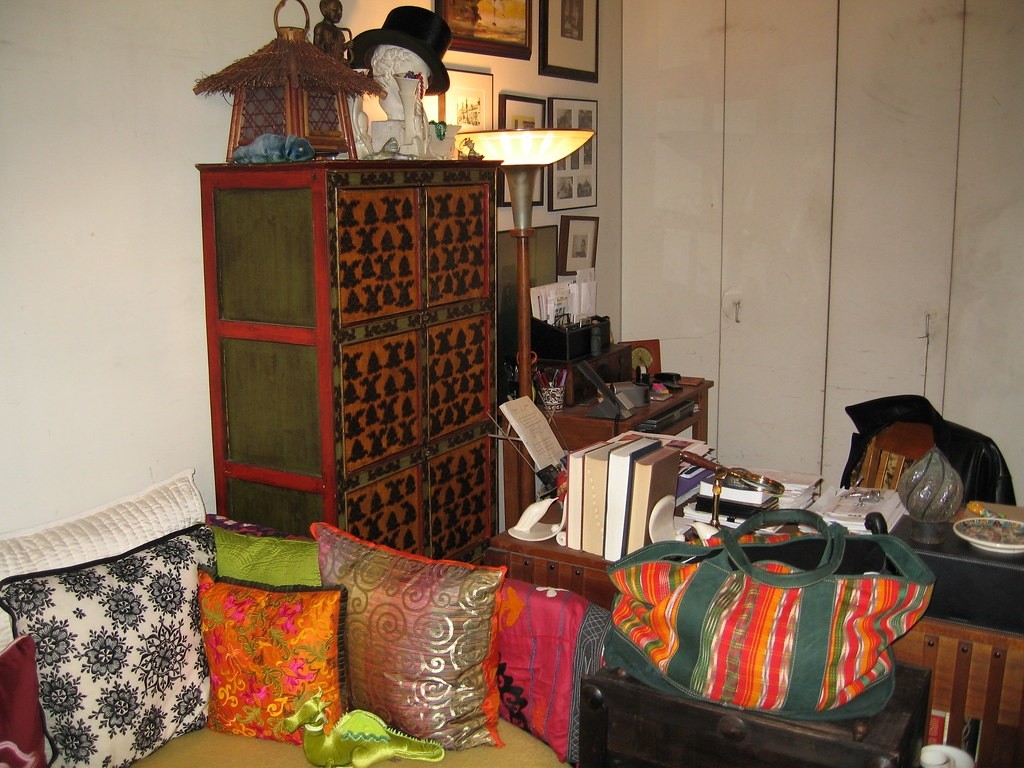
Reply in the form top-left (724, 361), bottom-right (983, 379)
top-left (602, 508), bottom-right (937, 721)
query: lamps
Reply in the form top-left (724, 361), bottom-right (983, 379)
top-left (449, 126), bottom-right (593, 503)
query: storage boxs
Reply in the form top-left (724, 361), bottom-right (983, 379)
top-left (497, 311), bottom-right (632, 399)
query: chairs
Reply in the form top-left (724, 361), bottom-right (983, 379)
top-left (838, 395), bottom-right (1018, 505)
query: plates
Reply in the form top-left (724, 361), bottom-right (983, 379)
top-left (953, 517), bottom-right (1024, 554)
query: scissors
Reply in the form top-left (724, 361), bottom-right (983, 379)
top-left (516, 351), bottom-right (537, 365)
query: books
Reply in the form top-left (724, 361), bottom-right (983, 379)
top-left (566, 430), bottom-right (717, 561)
top-left (695, 491), bottom-right (780, 520)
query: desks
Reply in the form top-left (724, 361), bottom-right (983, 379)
top-left (518, 375), bottom-right (716, 509)
top-left (490, 481), bottom-right (1024, 768)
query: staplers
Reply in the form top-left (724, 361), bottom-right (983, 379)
top-left (653, 372), bottom-right (683, 393)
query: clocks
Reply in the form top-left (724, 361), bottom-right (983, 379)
top-left (617, 339), bottom-right (661, 380)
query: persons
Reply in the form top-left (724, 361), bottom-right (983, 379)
top-left (371, 45), bottom-right (431, 120)
top-left (313, 0), bottom-right (353, 59)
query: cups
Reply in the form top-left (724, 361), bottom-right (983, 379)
top-left (540, 382), bottom-right (565, 412)
top-left (508, 378), bottom-right (536, 402)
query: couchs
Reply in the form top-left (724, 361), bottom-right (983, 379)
top-left (0, 464), bottom-right (606, 768)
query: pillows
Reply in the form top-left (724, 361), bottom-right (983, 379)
top-left (306, 522), bottom-right (513, 753)
top-left (0, 524), bottom-right (216, 768)
top-left (191, 571), bottom-right (350, 750)
top-left (186, 514), bottom-right (323, 589)
top-left (0, 635), bottom-right (48, 768)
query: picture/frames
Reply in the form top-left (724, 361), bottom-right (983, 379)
top-left (432, 0), bottom-right (532, 63)
top-left (547, 98), bottom-right (599, 213)
top-left (496, 94), bottom-right (546, 206)
top-left (535, 0), bottom-right (599, 84)
top-left (556, 216), bottom-right (600, 276)
top-left (438, 71), bottom-right (494, 133)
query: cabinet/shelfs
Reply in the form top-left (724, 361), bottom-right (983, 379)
top-left (190, 160), bottom-right (497, 568)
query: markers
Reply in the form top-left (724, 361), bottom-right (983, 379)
top-left (532, 368), bottom-right (567, 387)
